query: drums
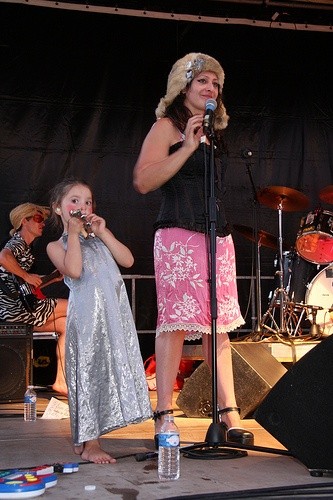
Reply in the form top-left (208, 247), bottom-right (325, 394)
top-left (303, 263), bottom-right (333, 336)
top-left (293, 207), bottom-right (333, 265)
top-left (271, 249), bottom-right (320, 313)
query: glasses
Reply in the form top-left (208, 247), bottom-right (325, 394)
top-left (33, 214), bottom-right (45, 223)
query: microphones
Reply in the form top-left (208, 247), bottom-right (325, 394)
top-left (202, 99), bottom-right (217, 132)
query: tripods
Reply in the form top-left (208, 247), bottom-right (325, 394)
top-left (135, 111), bottom-right (291, 463)
top-left (254, 197), bottom-right (304, 337)
top-left (290, 301), bottom-right (333, 340)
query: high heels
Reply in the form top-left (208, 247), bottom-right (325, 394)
top-left (152, 409), bottom-right (181, 451)
top-left (217, 407), bottom-right (255, 446)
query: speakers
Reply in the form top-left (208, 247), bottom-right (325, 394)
top-left (253, 334), bottom-right (333, 471)
top-left (176, 341), bottom-right (288, 420)
top-left (0, 321), bottom-right (33, 404)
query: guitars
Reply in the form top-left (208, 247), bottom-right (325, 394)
top-left (13, 271), bottom-right (61, 313)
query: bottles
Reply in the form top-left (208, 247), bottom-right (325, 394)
top-left (24, 385), bottom-right (37, 421)
top-left (157, 414), bottom-right (180, 480)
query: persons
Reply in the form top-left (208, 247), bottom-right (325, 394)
top-left (134, 52), bottom-right (254, 449)
top-left (46, 179), bottom-right (152, 464)
top-left (0, 202), bottom-right (77, 397)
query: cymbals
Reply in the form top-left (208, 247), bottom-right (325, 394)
top-left (318, 184), bottom-right (333, 204)
top-left (232, 223), bottom-right (290, 251)
top-left (256, 185), bottom-right (314, 213)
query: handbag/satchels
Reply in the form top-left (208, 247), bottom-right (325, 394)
top-left (143, 354), bottom-right (194, 391)
